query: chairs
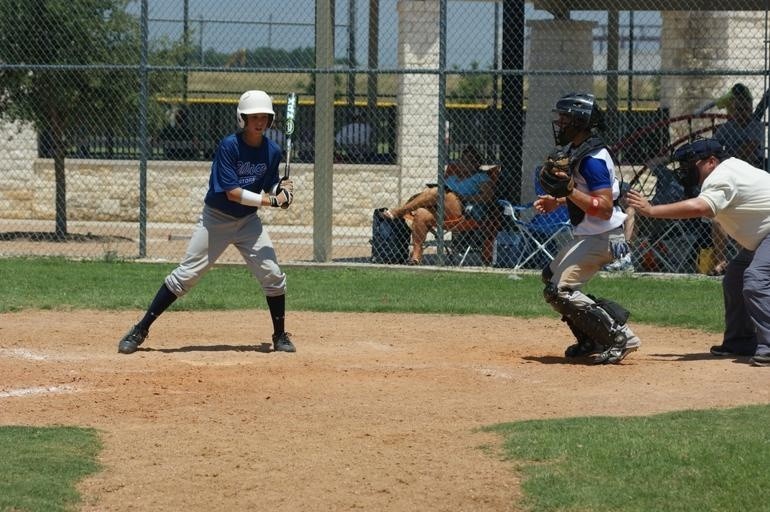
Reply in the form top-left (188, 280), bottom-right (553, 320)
top-left (405, 163), bottom-right (574, 270)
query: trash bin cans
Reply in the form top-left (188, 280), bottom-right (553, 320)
top-left (373, 207), bottom-right (409, 264)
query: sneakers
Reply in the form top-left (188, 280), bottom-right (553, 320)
top-left (707, 258), bottom-right (729, 276)
top-left (601, 252), bottom-right (635, 273)
top-left (565, 336), bottom-right (641, 365)
top-left (272, 332), bottom-right (296, 352)
top-left (118, 325), bottom-right (149, 354)
top-left (751, 355), bottom-right (770, 366)
top-left (710, 346), bottom-right (734, 356)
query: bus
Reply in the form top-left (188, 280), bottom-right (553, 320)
top-left (157, 98), bottom-right (666, 164)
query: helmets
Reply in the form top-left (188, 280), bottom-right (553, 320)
top-left (552, 92), bottom-right (605, 147)
top-left (237, 89), bottom-right (276, 129)
top-left (672, 139), bottom-right (731, 197)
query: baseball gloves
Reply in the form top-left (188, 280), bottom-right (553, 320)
top-left (539, 145), bottom-right (575, 199)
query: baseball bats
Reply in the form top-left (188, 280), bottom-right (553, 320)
top-left (280, 93), bottom-right (297, 209)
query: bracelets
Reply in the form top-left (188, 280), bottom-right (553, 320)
top-left (587, 196), bottom-right (600, 216)
top-left (239, 188), bottom-right (262, 208)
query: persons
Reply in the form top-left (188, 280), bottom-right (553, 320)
top-left (624, 137), bottom-right (770, 366)
top-left (712, 83), bottom-right (764, 170)
top-left (601, 145), bottom-right (709, 272)
top-left (118, 89), bottom-right (298, 353)
top-left (155, 107), bottom-right (199, 160)
top-left (532, 91), bottom-right (640, 364)
top-left (384, 147), bottom-right (496, 265)
top-left (615, 179), bottom-right (635, 256)
